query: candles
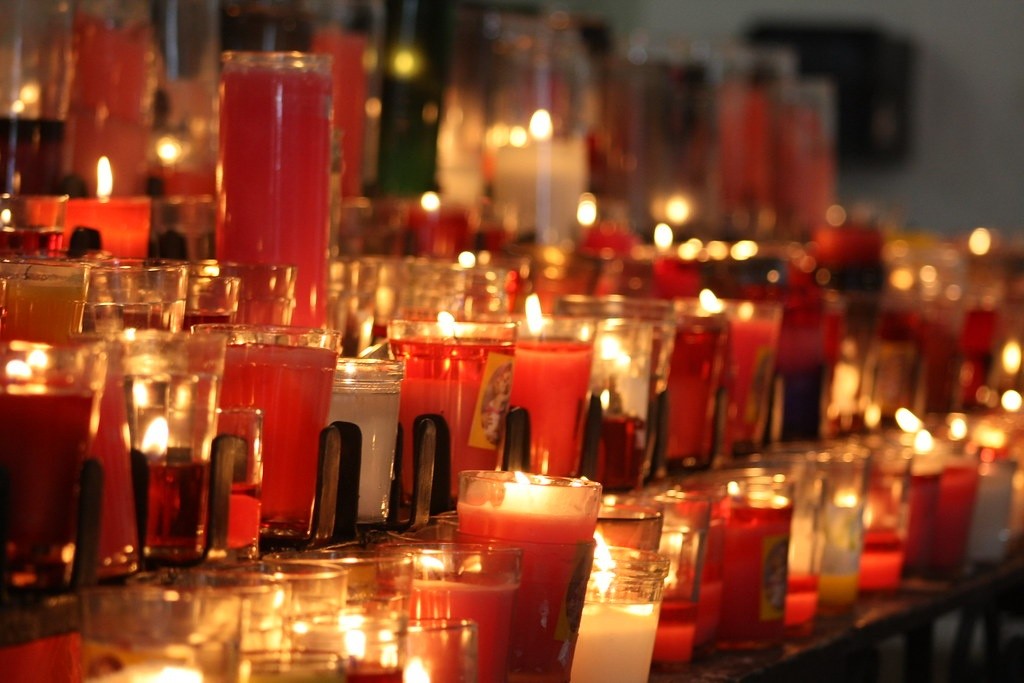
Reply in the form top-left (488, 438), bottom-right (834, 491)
top-left (0, 0), bottom-right (1024, 683)
